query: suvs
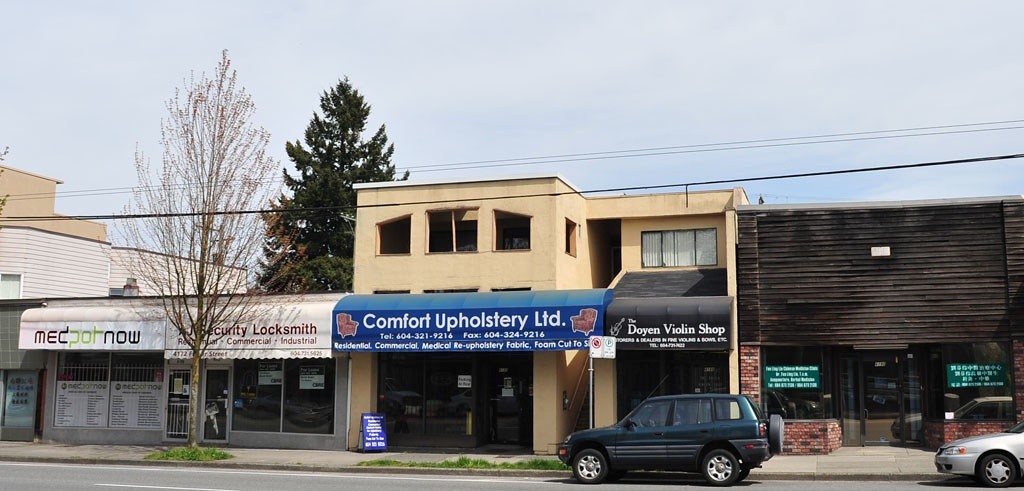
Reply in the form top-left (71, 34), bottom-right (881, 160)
top-left (702, 384), bottom-right (797, 420)
top-left (558, 393), bottom-right (784, 488)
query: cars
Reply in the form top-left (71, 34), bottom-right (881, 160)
top-left (891, 396), bottom-right (1013, 444)
top-left (934, 420), bottom-right (1023, 487)
top-left (387, 391), bottom-right (447, 419)
top-left (252, 393), bottom-right (281, 414)
top-left (449, 388), bottom-right (473, 421)
top-left (490, 395), bottom-right (522, 442)
